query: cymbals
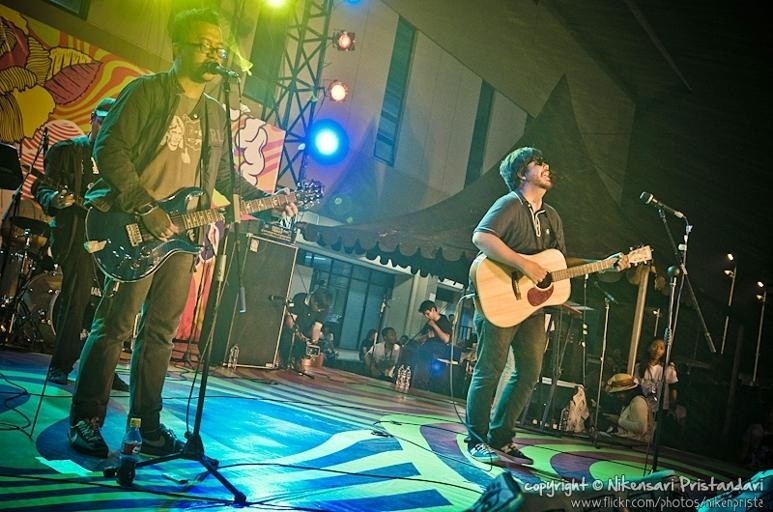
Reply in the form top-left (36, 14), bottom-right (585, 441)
top-left (7, 215), bottom-right (50, 237)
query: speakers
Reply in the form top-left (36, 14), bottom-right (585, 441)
top-left (197, 230), bottom-right (299, 370)
top-left (520, 376), bottom-right (590, 434)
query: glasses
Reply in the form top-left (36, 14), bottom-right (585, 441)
top-left (189, 40), bottom-right (228, 60)
top-left (529, 158), bottom-right (547, 166)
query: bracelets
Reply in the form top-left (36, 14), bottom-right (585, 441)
top-left (132, 201), bottom-right (160, 216)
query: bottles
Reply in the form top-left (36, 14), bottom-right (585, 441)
top-left (395, 363), bottom-right (411, 394)
top-left (558, 404), bottom-right (570, 431)
top-left (227, 343), bottom-right (240, 371)
top-left (113, 417), bottom-right (143, 488)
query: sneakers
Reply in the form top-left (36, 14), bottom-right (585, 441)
top-left (487, 443), bottom-right (534, 465)
top-left (68, 416), bottom-right (109, 459)
top-left (112, 374), bottom-right (130, 392)
top-left (126, 423), bottom-right (185, 454)
top-left (468, 442), bottom-right (501, 463)
top-left (49, 369), bottom-right (68, 385)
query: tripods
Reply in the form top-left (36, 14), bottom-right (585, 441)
top-left (271, 301), bottom-right (315, 380)
top-left (0, 237), bottom-right (44, 352)
top-left (104, 80), bottom-right (247, 508)
top-left (171, 258), bottom-right (206, 372)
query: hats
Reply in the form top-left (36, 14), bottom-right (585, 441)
top-left (94, 97), bottom-right (116, 117)
top-left (604, 373), bottom-right (638, 394)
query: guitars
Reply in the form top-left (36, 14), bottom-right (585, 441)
top-left (468, 239), bottom-right (652, 328)
top-left (21, 163), bottom-right (89, 213)
top-left (84, 179), bottom-right (325, 284)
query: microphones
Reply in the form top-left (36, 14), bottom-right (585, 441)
top-left (667, 266), bottom-right (680, 289)
top-left (269, 295), bottom-right (285, 301)
top-left (43, 129), bottom-right (48, 170)
top-left (640, 191), bottom-right (686, 220)
top-left (205, 61), bottom-right (240, 79)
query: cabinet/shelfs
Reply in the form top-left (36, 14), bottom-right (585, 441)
top-left (520, 307), bottom-right (573, 439)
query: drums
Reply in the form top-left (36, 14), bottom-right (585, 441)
top-left (1, 246), bottom-right (37, 305)
top-left (20, 267), bottom-right (103, 351)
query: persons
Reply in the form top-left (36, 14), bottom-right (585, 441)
top-left (273, 287), bottom-right (332, 378)
top-left (68, 6), bottom-right (299, 457)
top-left (36, 97), bottom-right (128, 389)
top-left (464, 147), bottom-right (632, 465)
top-left (359, 299), bottom-right (682, 445)
